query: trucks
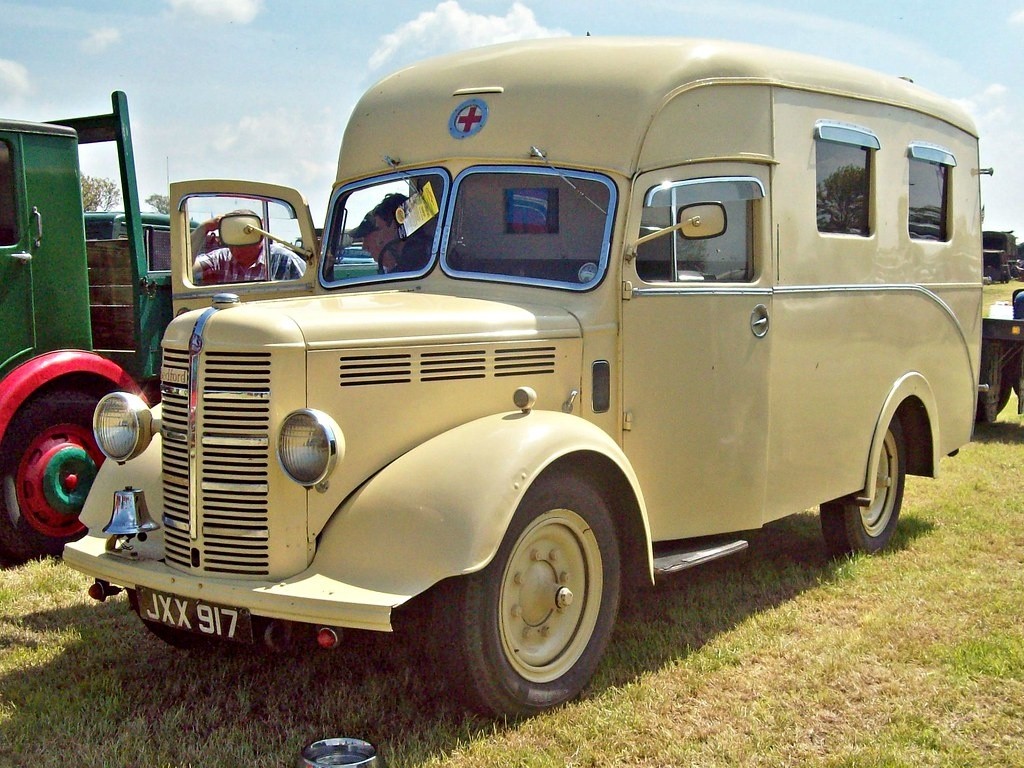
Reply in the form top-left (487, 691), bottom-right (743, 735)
top-left (0, 91), bottom-right (391, 568)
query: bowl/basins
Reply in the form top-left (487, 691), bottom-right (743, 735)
top-left (296, 738), bottom-right (379, 768)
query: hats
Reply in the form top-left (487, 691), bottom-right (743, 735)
top-left (349, 210), bottom-right (378, 238)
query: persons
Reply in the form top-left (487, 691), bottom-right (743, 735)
top-left (190, 209), bottom-right (306, 285)
top-left (348, 193), bottom-right (434, 274)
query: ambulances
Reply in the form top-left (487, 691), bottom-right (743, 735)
top-left (55, 31), bottom-right (990, 731)
